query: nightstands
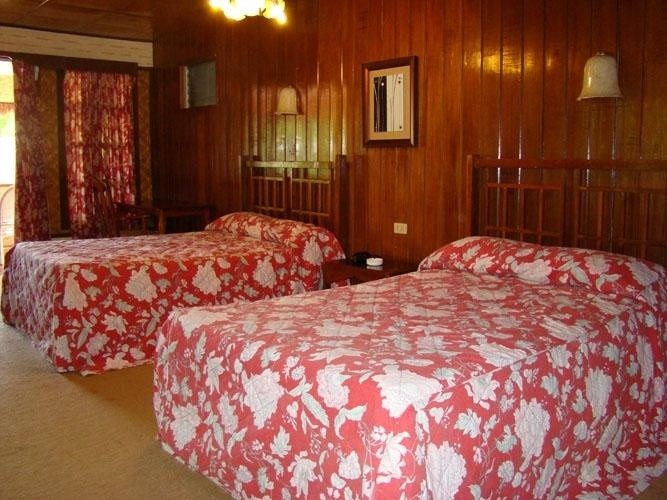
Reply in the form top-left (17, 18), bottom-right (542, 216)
top-left (323, 255), bottom-right (419, 291)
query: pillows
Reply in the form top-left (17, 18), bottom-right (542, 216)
top-left (204, 211), bottom-right (337, 260)
top-left (420, 236), bottom-right (667, 313)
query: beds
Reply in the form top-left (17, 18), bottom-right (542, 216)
top-left (2, 155), bottom-right (348, 378)
top-left (153, 152), bottom-right (667, 500)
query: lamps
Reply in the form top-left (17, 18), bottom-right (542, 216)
top-left (274, 85), bottom-right (299, 116)
top-left (577, 49), bottom-right (622, 100)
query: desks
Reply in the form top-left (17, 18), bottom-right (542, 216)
top-left (114, 200), bottom-right (211, 235)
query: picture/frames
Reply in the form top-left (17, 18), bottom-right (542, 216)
top-left (362, 55), bottom-right (416, 147)
